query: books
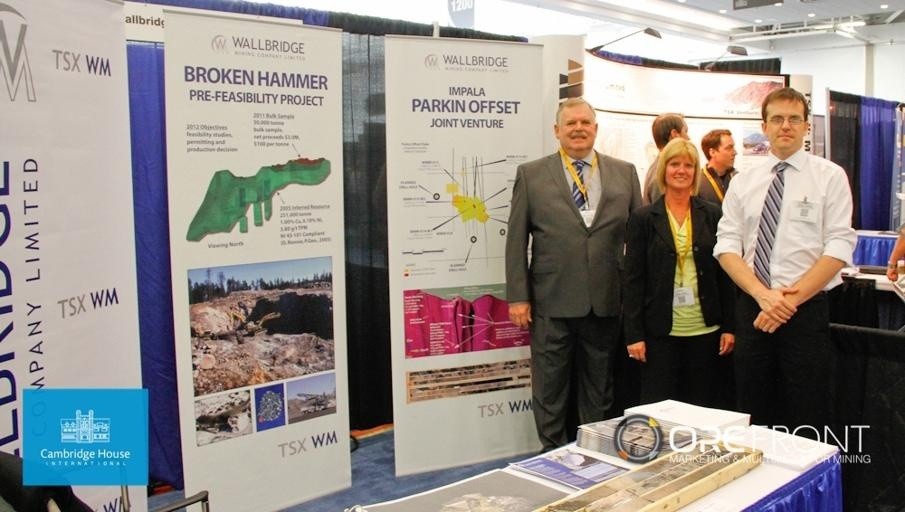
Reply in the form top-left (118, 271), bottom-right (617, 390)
top-left (575, 398), bottom-right (751, 458)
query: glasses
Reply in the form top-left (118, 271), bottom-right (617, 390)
top-left (765, 116), bottom-right (805, 124)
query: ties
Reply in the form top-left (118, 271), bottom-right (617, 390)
top-left (572, 160), bottom-right (586, 210)
top-left (754, 162), bottom-right (788, 291)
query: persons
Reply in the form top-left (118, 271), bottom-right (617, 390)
top-left (886, 221), bottom-right (905, 282)
top-left (506, 95), bottom-right (645, 454)
top-left (622, 112), bottom-right (739, 406)
top-left (712, 86), bottom-right (858, 442)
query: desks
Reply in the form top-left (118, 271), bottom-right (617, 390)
top-left (348, 402), bottom-right (844, 512)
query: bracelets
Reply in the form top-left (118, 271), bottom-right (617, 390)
top-left (886, 264), bottom-right (897, 269)
